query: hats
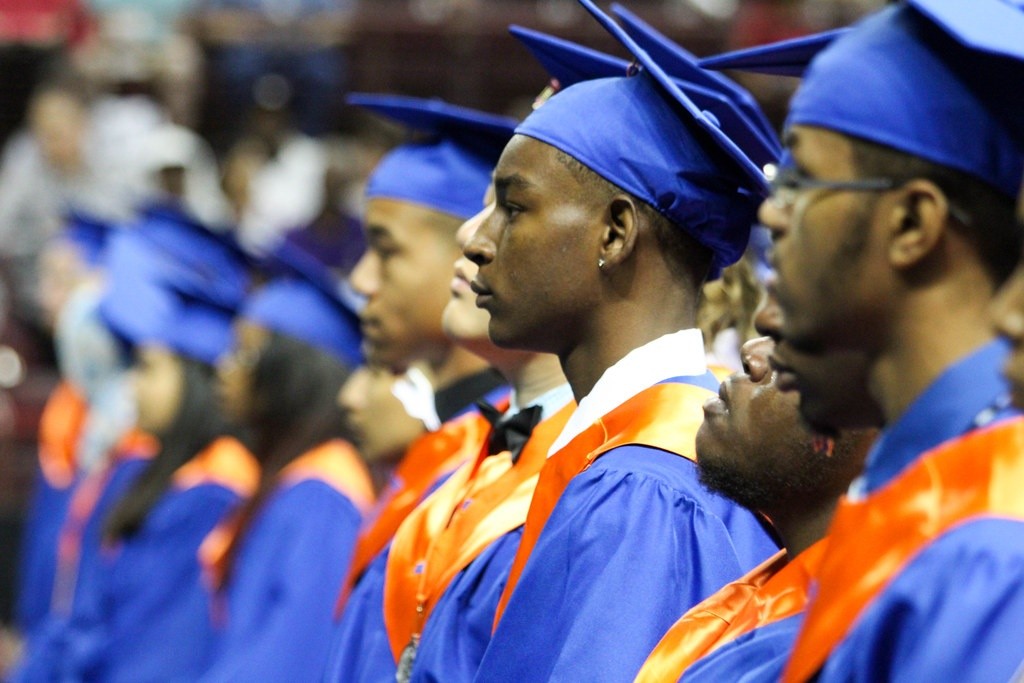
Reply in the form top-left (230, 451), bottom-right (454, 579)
top-left (507, 0), bottom-right (1024, 275)
top-left (346, 90), bottom-right (522, 220)
top-left (65, 199), bottom-right (369, 366)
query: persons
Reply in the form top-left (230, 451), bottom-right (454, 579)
top-left (1, 0), bottom-right (1023, 682)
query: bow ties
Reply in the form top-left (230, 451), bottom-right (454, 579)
top-left (473, 399), bottom-right (543, 464)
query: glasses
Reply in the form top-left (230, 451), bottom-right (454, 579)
top-left (763, 160), bottom-right (968, 225)
